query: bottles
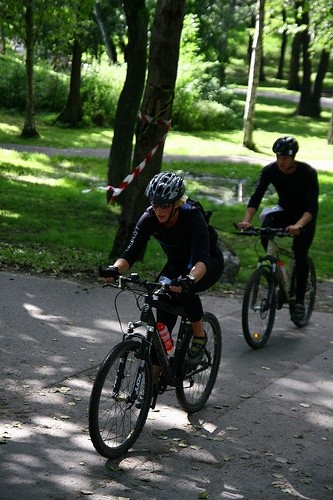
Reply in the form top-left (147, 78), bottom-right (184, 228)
top-left (278, 260), bottom-right (288, 281)
top-left (157, 322), bottom-right (174, 354)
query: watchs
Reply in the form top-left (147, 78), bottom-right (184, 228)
top-left (186, 274), bottom-right (195, 285)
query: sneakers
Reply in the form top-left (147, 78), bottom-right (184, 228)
top-left (292, 304), bottom-right (305, 320)
top-left (187, 329), bottom-right (207, 368)
top-left (135, 382), bottom-right (156, 409)
top-left (264, 272), bottom-right (278, 289)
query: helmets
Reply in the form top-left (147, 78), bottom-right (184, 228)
top-left (273, 138), bottom-right (298, 156)
top-left (145, 171), bottom-right (185, 202)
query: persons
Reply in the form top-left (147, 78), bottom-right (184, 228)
top-left (237, 136), bottom-right (319, 321)
top-left (102, 171), bottom-right (224, 409)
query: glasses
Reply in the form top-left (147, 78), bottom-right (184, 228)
top-left (151, 201), bottom-right (174, 209)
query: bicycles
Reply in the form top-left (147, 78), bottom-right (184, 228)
top-left (231, 221), bottom-right (318, 349)
top-left (86, 272), bottom-right (222, 459)
